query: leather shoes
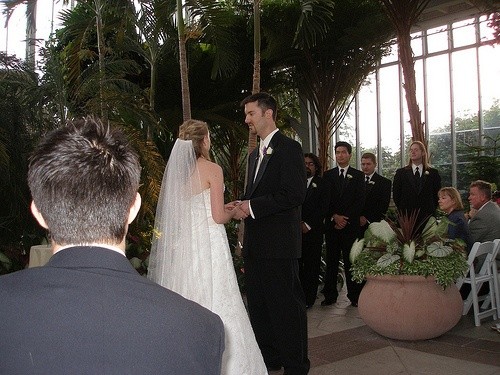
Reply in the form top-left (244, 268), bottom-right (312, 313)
top-left (305, 299), bottom-right (359, 311)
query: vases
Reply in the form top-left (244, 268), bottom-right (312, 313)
top-left (357, 273), bottom-right (463, 341)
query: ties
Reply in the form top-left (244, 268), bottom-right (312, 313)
top-left (366, 176), bottom-right (370, 190)
top-left (254, 143), bottom-right (265, 182)
top-left (339, 169), bottom-right (346, 191)
top-left (415, 167), bottom-right (421, 180)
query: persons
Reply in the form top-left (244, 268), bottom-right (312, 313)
top-left (437, 185), bottom-right (480, 278)
top-left (230, 92), bottom-right (313, 375)
top-left (463, 190), bottom-right (500, 221)
top-left (320, 141), bottom-right (367, 308)
top-left (146, 118), bottom-right (270, 374)
top-left (1, 113), bottom-right (226, 375)
top-left (356, 152), bottom-right (393, 240)
top-left (302, 151), bottom-right (325, 310)
top-left (468, 180), bottom-right (500, 276)
top-left (393, 139), bottom-right (442, 240)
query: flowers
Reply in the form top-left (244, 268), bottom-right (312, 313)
top-left (346, 206), bottom-right (470, 288)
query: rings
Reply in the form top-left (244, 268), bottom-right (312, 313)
top-left (342, 224), bottom-right (344, 226)
top-left (240, 216), bottom-right (244, 221)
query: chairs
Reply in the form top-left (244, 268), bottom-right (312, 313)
top-left (480, 238), bottom-right (500, 317)
top-left (454, 242), bottom-right (498, 327)
